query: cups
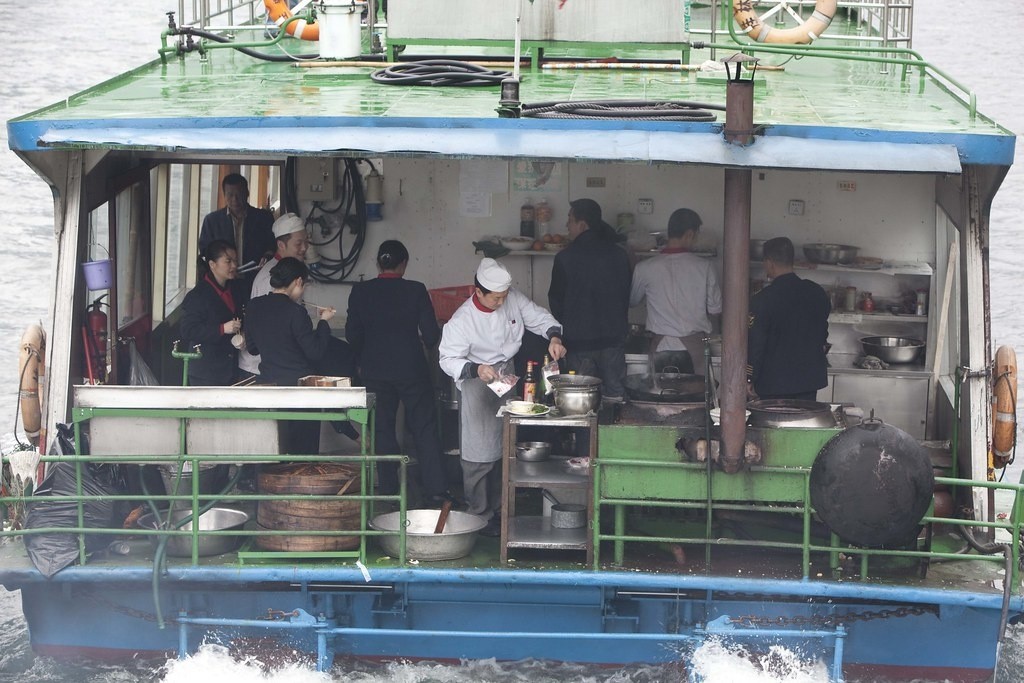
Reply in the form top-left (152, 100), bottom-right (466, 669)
top-left (231, 335), bottom-right (244, 350)
top-left (618, 213), bottom-right (635, 232)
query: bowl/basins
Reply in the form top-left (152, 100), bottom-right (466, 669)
top-left (548, 374), bottom-right (603, 415)
top-left (500, 236), bottom-right (536, 251)
top-left (858, 336), bottom-right (925, 363)
top-left (628, 323), bottom-right (655, 339)
top-left (708, 338), bottom-right (723, 357)
top-left (369, 502), bottom-right (488, 561)
top-left (751, 239), bottom-right (768, 259)
top-left (514, 441), bottom-right (553, 462)
top-left (136, 508), bottom-right (248, 557)
top-left (802, 244), bottom-right (860, 264)
top-left (545, 243), bottom-right (569, 253)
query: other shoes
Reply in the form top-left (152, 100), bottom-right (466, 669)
top-left (382, 491), bottom-right (417, 510)
top-left (479, 522), bottom-right (499, 536)
top-left (429, 491), bottom-right (462, 511)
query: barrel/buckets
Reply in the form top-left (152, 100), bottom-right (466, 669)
top-left (156, 465), bottom-right (218, 509)
top-left (80, 243), bottom-right (114, 290)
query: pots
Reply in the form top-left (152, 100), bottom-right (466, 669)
top-left (810, 418), bottom-right (934, 550)
top-left (541, 489), bottom-right (587, 529)
top-left (620, 366), bottom-right (719, 403)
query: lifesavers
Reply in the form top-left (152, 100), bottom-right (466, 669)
top-left (994, 343), bottom-right (1017, 470)
top-left (729, 3), bottom-right (837, 48)
top-left (265, 0), bottom-right (365, 41)
top-left (19, 324), bottom-right (45, 446)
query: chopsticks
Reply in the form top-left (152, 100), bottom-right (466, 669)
top-left (232, 317), bottom-right (240, 336)
top-left (301, 301), bottom-right (337, 313)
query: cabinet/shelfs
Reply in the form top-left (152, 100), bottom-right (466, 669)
top-left (498, 411), bottom-right (597, 566)
top-left (494, 238), bottom-right (930, 325)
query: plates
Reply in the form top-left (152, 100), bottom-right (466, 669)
top-left (505, 401), bottom-right (550, 417)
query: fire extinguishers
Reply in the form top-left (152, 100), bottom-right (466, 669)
top-left (85, 293), bottom-right (111, 387)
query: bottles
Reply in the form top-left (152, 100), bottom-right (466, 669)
top-left (537, 198), bottom-right (552, 241)
top-left (520, 198), bottom-right (534, 237)
top-left (523, 354), bottom-right (553, 406)
top-left (844, 286), bottom-right (929, 316)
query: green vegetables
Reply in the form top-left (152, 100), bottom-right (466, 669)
top-left (532, 404), bottom-right (549, 413)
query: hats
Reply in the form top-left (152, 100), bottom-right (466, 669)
top-left (476, 257), bottom-right (512, 293)
top-left (272, 211), bottom-right (305, 238)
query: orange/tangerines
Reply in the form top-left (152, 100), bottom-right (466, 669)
top-left (543, 234), bottom-right (562, 243)
top-left (533, 242), bottom-right (542, 250)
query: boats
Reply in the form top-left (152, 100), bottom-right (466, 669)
top-left (0, 0), bottom-right (1023, 683)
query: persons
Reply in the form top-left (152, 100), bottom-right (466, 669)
top-left (548, 199), bottom-right (638, 399)
top-left (629, 208), bottom-right (723, 374)
top-left (183, 173), bottom-right (370, 461)
top-left (344, 241), bottom-right (444, 501)
top-left (439, 256), bottom-right (567, 537)
top-left (748, 236), bottom-right (830, 401)
